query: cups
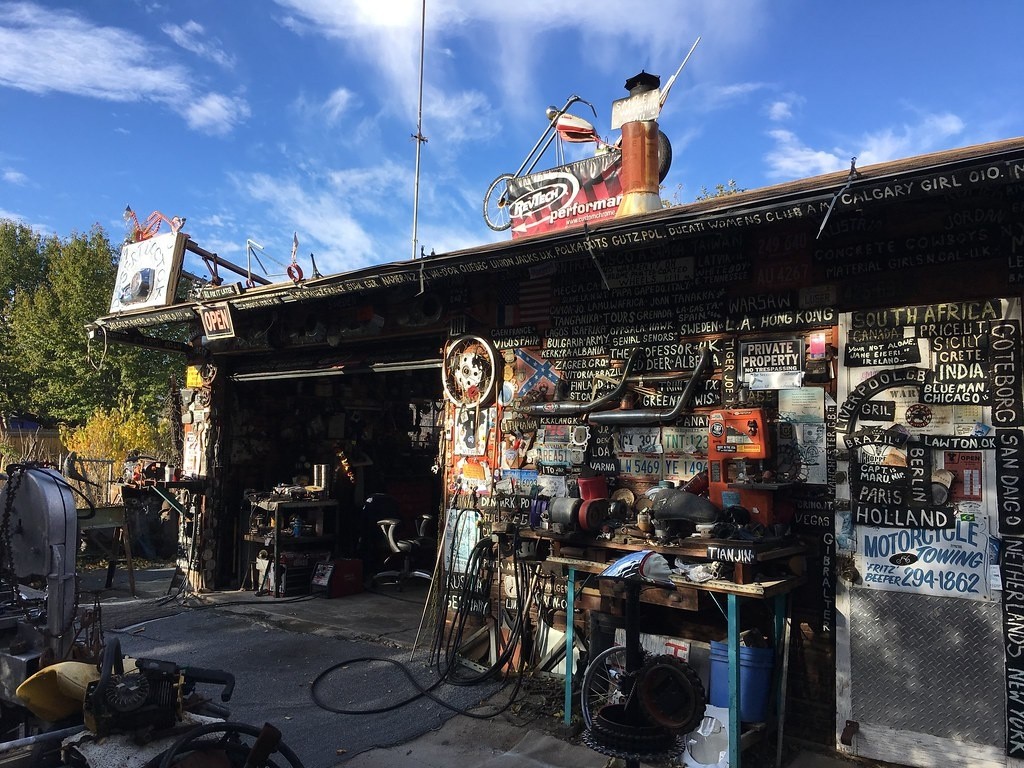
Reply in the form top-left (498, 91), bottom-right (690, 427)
top-left (165, 465), bottom-right (175, 482)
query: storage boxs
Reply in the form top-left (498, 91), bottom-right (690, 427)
top-left (607, 628), bottom-right (711, 705)
top-left (255, 558), bottom-right (313, 596)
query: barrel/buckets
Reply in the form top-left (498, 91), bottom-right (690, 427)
top-left (709, 642), bottom-right (781, 725)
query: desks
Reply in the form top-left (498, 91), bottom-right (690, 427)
top-left (77, 505), bottom-right (136, 597)
top-left (535, 529), bottom-right (808, 768)
top-left (237, 497), bottom-right (341, 598)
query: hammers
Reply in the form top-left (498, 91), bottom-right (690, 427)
top-left (255, 557), bottom-right (274, 597)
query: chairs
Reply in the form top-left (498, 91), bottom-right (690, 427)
top-left (360, 492), bottom-right (433, 593)
